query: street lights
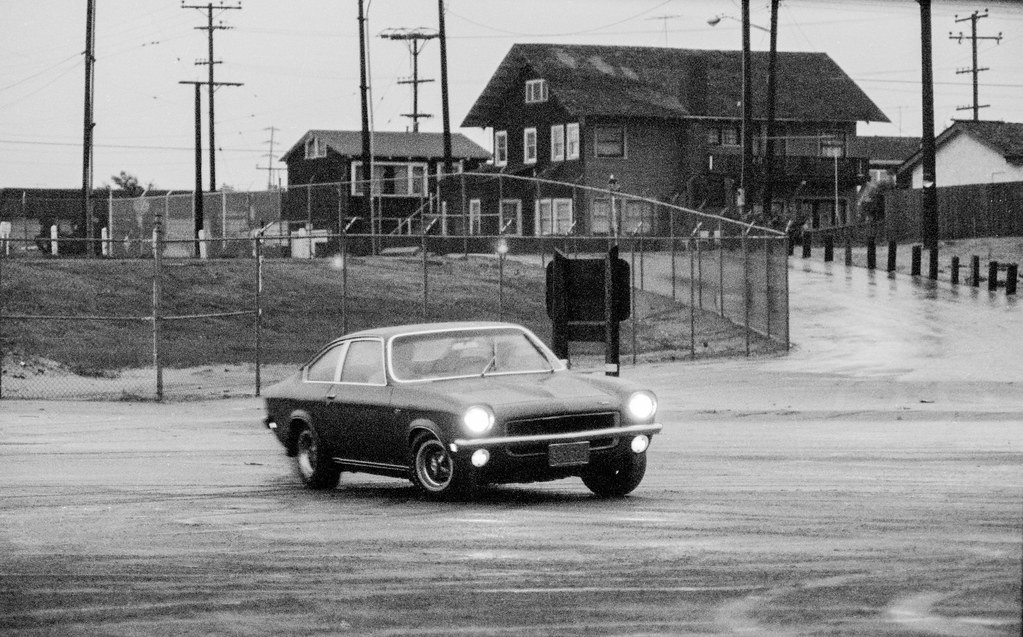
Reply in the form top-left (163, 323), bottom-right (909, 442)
top-left (705, 15), bottom-right (777, 224)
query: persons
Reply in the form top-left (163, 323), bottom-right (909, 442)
top-left (367, 343), bottom-right (427, 386)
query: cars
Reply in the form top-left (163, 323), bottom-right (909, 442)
top-left (258, 321), bottom-right (664, 503)
